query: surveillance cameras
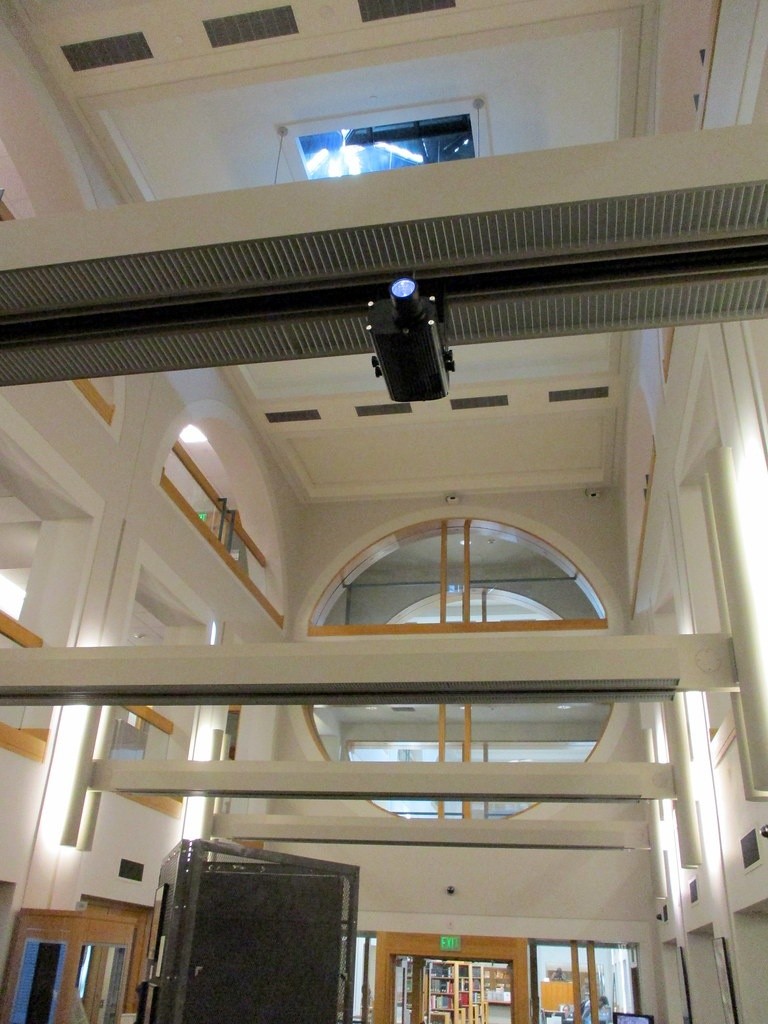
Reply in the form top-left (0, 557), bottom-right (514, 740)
top-left (760, 826), bottom-right (768, 838)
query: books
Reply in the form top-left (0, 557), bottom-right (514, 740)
top-left (459, 979), bottom-right (469, 991)
top-left (473, 992), bottom-right (481, 1002)
top-left (431, 979), bottom-right (453, 993)
top-left (459, 994), bottom-right (469, 1005)
top-left (406, 965), bottom-right (412, 1006)
top-left (484, 970), bottom-right (510, 978)
top-left (431, 964), bottom-right (455, 977)
top-left (431, 994), bottom-right (453, 1009)
top-left (473, 979), bottom-right (480, 989)
top-left (459, 967), bottom-right (468, 977)
top-left (472, 967), bottom-right (481, 977)
top-left (484, 980), bottom-right (510, 988)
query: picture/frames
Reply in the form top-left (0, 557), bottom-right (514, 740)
top-left (712, 936), bottom-right (740, 1024)
top-left (676, 946), bottom-right (693, 1024)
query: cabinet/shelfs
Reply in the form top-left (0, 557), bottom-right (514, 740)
top-left (483, 967), bottom-right (511, 1005)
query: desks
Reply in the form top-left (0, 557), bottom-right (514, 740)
top-left (428, 961), bottom-right (489, 1024)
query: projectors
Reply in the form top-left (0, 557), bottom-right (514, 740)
top-left (367, 278), bottom-right (451, 402)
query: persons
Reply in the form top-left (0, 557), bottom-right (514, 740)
top-left (563, 1005), bottom-right (572, 1018)
top-left (582, 995), bottom-right (610, 1021)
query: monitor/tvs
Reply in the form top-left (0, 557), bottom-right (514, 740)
top-left (613, 1012), bottom-right (655, 1024)
